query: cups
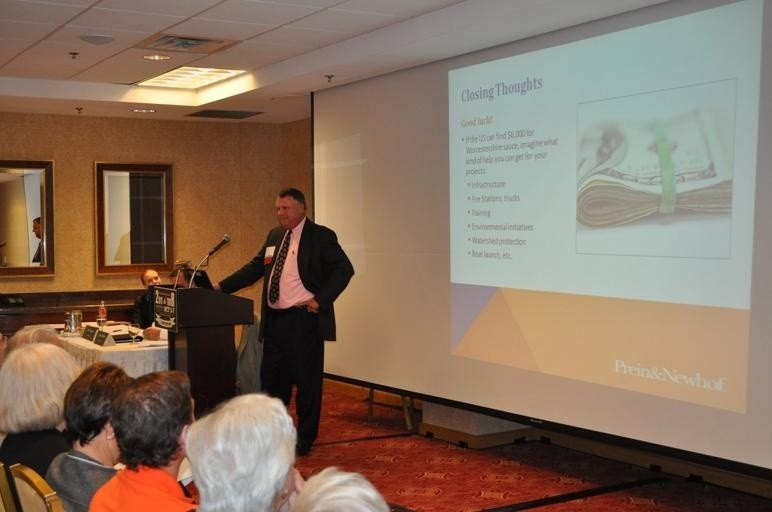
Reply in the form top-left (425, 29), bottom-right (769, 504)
top-left (97, 318), bottom-right (107, 332)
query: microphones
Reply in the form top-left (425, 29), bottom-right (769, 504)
top-left (0, 242), bottom-right (7, 248)
top-left (208, 234), bottom-right (231, 256)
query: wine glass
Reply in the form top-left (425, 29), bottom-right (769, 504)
top-left (128, 324), bottom-right (140, 349)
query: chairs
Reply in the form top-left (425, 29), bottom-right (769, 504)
top-left (0, 460), bottom-right (17, 512)
top-left (9, 463), bottom-right (64, 512)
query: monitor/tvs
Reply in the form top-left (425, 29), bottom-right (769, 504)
top-left (187, 269), bottom-right (213, 290)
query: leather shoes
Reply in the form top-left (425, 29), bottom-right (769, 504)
top-left (297, 439), bottom-right (311, 454)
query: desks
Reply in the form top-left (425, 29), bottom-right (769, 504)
top-left (25, 322), bottom-right (168, 378)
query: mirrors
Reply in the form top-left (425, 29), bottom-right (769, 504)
top-left (94, 160), bottom-right (176, 277)
top-left (0, 160), bottom-right (57, 278)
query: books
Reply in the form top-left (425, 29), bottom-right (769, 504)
top-left (177, 455), bottom-right (196, 487)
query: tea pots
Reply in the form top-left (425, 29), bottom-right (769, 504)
top-left (62, 310), bottom-right (83, 333)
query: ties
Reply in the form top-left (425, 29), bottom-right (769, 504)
top-left (269, 229), bottom-right (293, 305)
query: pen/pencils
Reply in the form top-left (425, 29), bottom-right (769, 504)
top-left (113, 329), bottom-right (121, 332)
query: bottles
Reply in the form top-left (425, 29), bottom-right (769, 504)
top-left (98, 303), bottom-right (106, 318)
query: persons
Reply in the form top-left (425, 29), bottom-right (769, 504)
top-left (2, 342), bottom-right (83, 512)
top-left (43, 363), bottom-right (138, 512)
top-left (285, 464), bottom-right (390, 511)
top-left (131, 269), bottom-right (163, 330)
top-left (214, 188), bottom-right (355, 457)
top-left (89, 370), bottom-right (197, 512)
top-left (184, 394), bottom-right (304, 512)
top-left (31, 214), bottom-right (44, 266)
top-left (142, 267), bottom-right (192, 342)
top-left (8, 325), bottom-right (67, 355)
